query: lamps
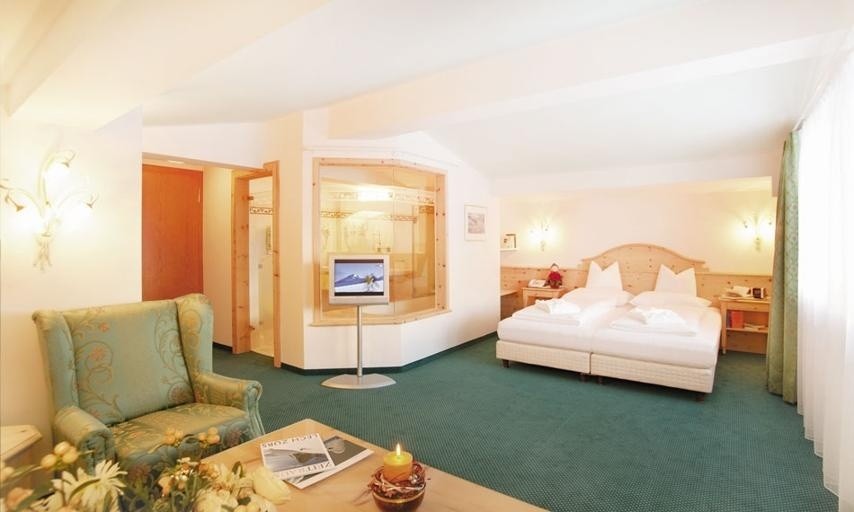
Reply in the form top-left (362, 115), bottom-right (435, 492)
top-left (738, 213), bottom-right (773, 255)
top-left (527, 218), bottom-right (559, 251)
top-left (0, 152), bottom-right (114, 275)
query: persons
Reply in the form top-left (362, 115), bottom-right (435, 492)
top-left (364, 273), bottom-right (376, 290)
top-left (369, 273), bottom-right (381, 288)
top-left (543, 262), bottom-right (563, 289)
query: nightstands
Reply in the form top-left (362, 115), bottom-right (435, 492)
top-left (722, 293), bottom-right (770, 354)
top-left (524, 285), bottom-right (563, 308)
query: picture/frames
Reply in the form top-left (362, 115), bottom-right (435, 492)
top-left (464, 203), bottom-right (488, 240)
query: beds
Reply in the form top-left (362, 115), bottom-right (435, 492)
top-left (496, 242), bottom-right (722, 398)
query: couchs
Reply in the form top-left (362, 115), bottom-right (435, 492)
top-left (30, 293), bottom-right (262, 480)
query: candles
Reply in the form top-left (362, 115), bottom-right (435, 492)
top-left (382, 443), bottom-right (413, 483)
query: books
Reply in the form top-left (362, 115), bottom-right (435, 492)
top-left (742, 321), bottom-right (767, 332)
top-left (257, 430), bottom-right (378, 493)
top-left (717, 285), bottom-right (751, 300)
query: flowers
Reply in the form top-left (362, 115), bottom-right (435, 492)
top-left (3, 440), bottom-right (272, 512)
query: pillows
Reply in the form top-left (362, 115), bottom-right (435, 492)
top-left (652, 263), bottom-right (698, 297)
top-left (585, 260), bottom-right (624, 290)
top-left (559, 287), bottom-right (634, 306)
top-left (631, 290), bottom-right (713, 317)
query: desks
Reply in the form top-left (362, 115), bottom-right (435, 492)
top-left (1, 423), bottom-right (41, 464)
top-left (162, 418), bottom-right (551, 512)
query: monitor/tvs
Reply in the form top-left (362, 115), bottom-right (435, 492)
top-left (329, 254), bottom-right (390, 305)
